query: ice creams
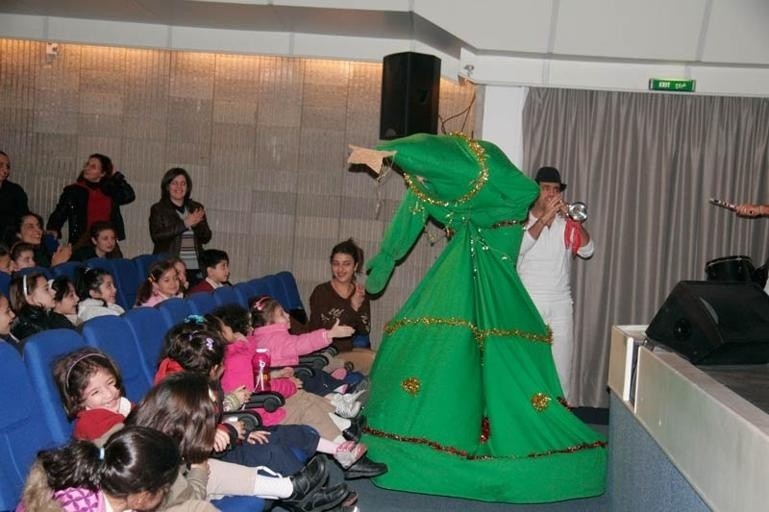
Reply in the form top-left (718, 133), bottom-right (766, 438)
top-left (549, 197), bottom-right (588, 222)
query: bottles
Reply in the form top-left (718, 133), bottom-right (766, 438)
top-left (534, 166), bottom-right (566, 193)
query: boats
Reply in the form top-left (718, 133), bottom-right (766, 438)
top-left (380, 52), bottom-right (441, 140)
top-left (645, 280), bottom-right (769, 363)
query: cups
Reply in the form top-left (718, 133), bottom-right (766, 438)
top-left (81, 160), bottom-right (100, 174)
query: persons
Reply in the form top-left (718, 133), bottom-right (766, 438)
top-left (308, 240), bottom-right (372, 443)
top-left (2, 153), bottom-right (136, 275)
top-left (513, 164), bottom-right (594, 406)
top-left (735, 202), bottom-right (769, 218)
top-left (2, 268), bottom-right (124, 353)
top-left (17, 295), bottom-right (386, 512)
top-left (130, 168), bottom-right (230, 308)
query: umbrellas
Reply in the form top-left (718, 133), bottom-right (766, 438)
top-left (294, 481), bottom-right (350, 512)
top-left (341, 491), bottom-right (358, 506)
top-left (342, 414), bottom-right (367, 444)
top-left (284, 454), bottom-right (328, 509)
top-left (348, 379), bottom-right (368, 394)
top-left (344, 455), bottom-right (387, 480)
top-left (327, 505), bottom-right (360, 512)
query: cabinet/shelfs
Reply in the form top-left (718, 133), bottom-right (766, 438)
top-left (250, 347), bottom-right (273, 393)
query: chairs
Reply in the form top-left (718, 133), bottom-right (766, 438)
top-left (0, 236), bottom-right (372, 512)
top-left (333, 442), bottom-right (365, 470)
top-left (342, 389), bottom-right (368, 418)
